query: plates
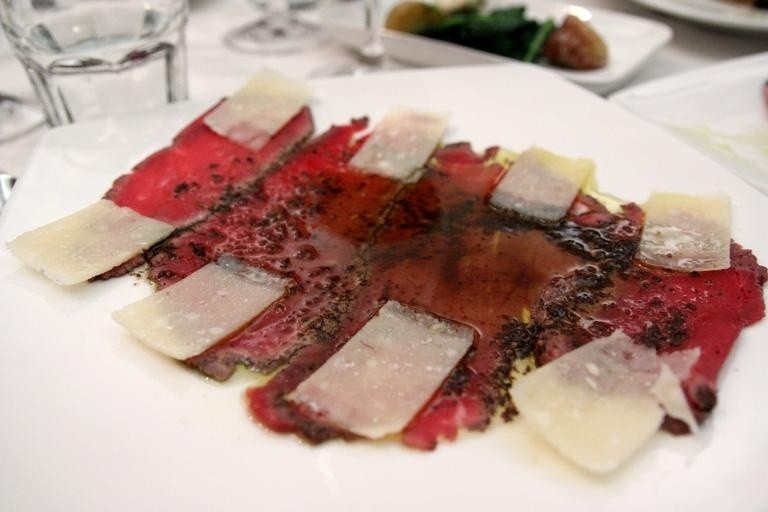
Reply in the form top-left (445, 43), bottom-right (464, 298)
top-left (314, 0), bottom-right (675, 99)
top-left (0, 58), bottom-right (768, 512)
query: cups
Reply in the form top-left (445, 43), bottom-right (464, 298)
top-left (0, 0), bottom-right (193, 130)
